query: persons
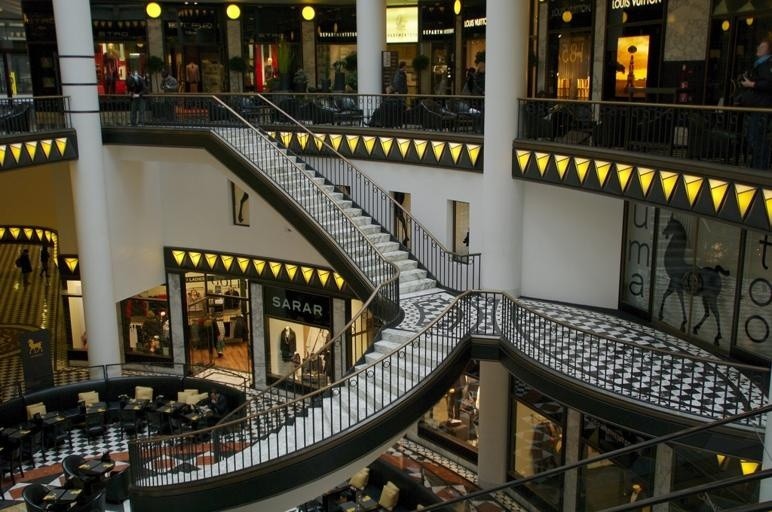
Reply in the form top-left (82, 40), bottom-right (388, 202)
top-left (20, 248), bottom-right (33, 285)
top-left (126, 70), bottom-right (145, 127)
top-left (433, 56), bottom-right (450, 95)
top-left (533, 90), bottom-right (563, 127)
top-left (467, 382), bottom-right (480, 447)
top-left (103, 42), bottom-right (120, 94)
top-left (160, 71), bottom-right (177, 121)
top-left (447, 374), bottom-right (467, 423)
top-left (720, 43), bottom-right (768, 167)
top-left (365, 86), bottom-right (399, 127)
top-left (279, 325), bottom-right (299, 381)
top-left (395, 62), bottom-right (407, 100)
top-left (39, 245), bottom-right (51, 277)
top-left (472, 62), bottom-right (484, 111)
top-left (198, 388), bottom-right (227, 433)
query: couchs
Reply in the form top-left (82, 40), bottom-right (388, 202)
top-left (320, 456), bottom-right (458, 512)
top-left (0, 375), bottom-right (246, 435)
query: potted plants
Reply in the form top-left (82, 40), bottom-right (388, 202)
top-left (144, 55), bottom-right (166, 118)
top-left (293, 69), bottom-right (309, 92)
top-left (332, 59), bottom-right (348, 91)
top-left (410, 55), bottom-right (428, 124)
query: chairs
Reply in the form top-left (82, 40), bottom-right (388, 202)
top-left (0, 415), bottom-right (131, 512)
top-left (0, 101), bottom-right (32, 133)
top-left (208, 89), bottom-right (484, 133)
top-left (522, 97), bottom-right (769, 168)
